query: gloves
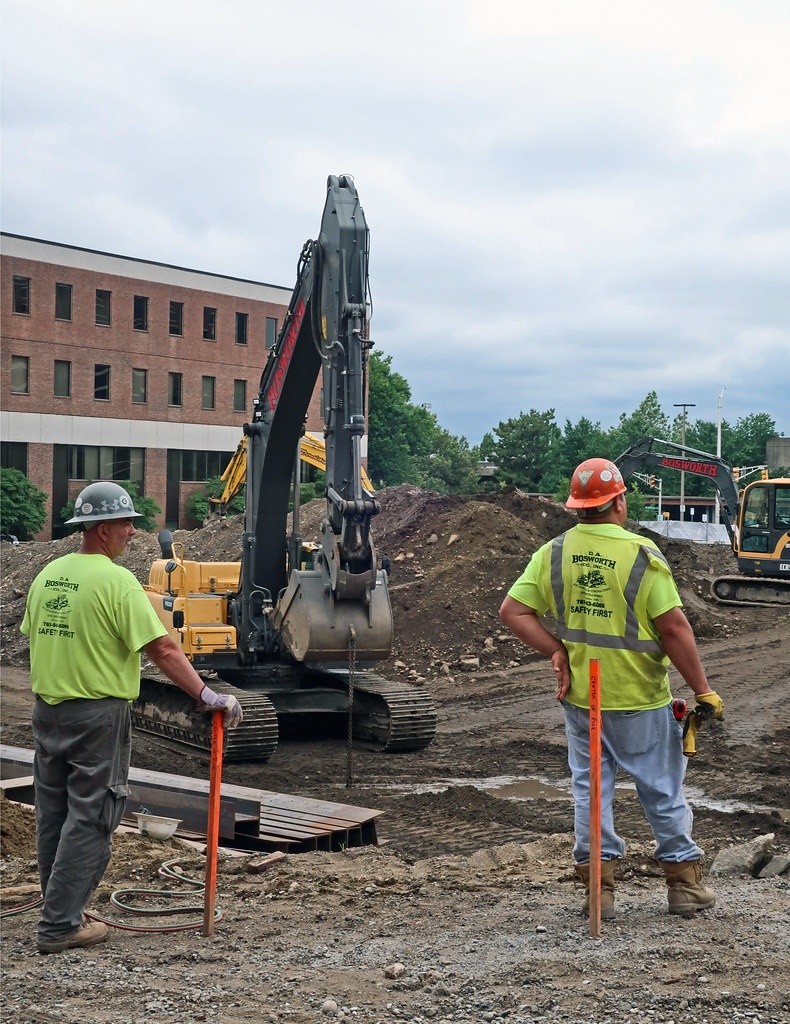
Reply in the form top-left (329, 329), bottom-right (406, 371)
top-left (682, 691), bottom-right (729, 757)
top-left (196, 686), bottom-right (243, 729)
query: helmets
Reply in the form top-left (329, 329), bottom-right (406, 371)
top-left (566, 459), bottom-right (627, 508)
top-left (64, 483), bottom-right (144, 524)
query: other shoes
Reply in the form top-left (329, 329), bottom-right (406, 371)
top-left (79, 909), bottom-right (100, 920)
top-left (38, 921), bottom-right (107, 952)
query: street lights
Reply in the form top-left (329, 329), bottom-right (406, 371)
top-left (672, 403), bottom-right (698, 521)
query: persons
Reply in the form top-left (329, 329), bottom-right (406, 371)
top-left (19, 482), bottom-right (243, 953)
top-left (501, 458), bottom-right (724, 919)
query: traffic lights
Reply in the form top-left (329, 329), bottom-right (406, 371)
top-left (733, 467), bottom-right (739, 482)
top-left (649, 475), bottom-right (656, 490)
top-left (761, 470), bottom-right (769, 480)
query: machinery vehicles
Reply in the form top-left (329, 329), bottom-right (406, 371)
top-left (127, 173), bottom-right (440, 768)
top-left (612, 434), bottom-right (790, 610)
top-left (201, 430), bottom-right (377, 531)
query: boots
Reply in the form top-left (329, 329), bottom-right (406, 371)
top-left (575, 861), bottom-right (616, 918)
top-left (663, 862), bottom-right (717, 914)
